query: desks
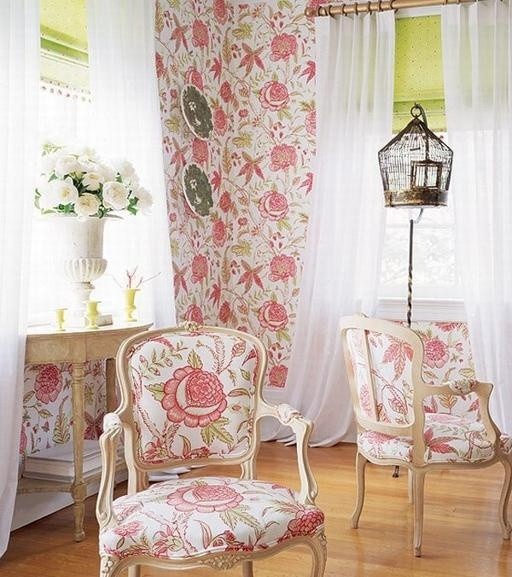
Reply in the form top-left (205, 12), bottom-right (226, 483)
top-left (14, 321), bottom-right (158, 541)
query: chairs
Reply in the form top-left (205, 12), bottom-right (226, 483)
top-left (93, 322), bottom-right (329, 576)
top-left (341, 318), bottom-right (511, 558)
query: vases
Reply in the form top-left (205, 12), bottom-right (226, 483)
top-left (53, 213), bottom-right (117, 327)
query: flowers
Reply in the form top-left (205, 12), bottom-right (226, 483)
top-left (28, 140), bottom-right (155, 222)
